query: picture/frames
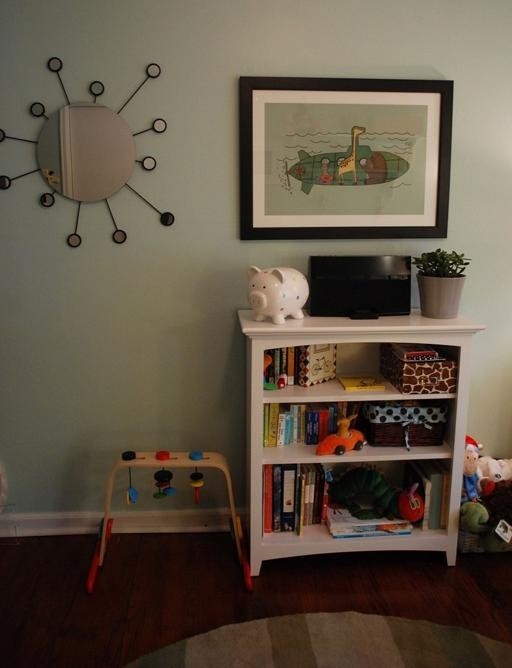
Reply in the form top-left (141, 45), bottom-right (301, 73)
top-left (238, 74), bottom-right (455, 240)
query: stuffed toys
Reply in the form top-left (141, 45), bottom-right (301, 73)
top-left (460, 434), bottom-right (512, 550)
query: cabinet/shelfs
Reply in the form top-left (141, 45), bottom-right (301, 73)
top-left (236, 309), bottom-right (487, 577)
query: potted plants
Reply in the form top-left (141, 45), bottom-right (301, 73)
top-left (411, 248), bottom-right (472, 319)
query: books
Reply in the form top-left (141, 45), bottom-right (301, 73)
top-left (328, 507), bottom-right (413, 538)
top-left (319, 403), bottom-right (363, 429)
top-left (263, 344), bottom-right (337, 390)
top-left (338, 375), bottom-right (386, 391)
top-left (262, 463), bottom-right (329, 536)
top-left (263, 401), bottom-right (319, 448)
top-left (392, 343), bottom-right (439, 361)
top-left (405, 462), bottom-right (451, 532)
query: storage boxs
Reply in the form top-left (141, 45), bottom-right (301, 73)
top-left (380, 350), bottom-right (457, 394)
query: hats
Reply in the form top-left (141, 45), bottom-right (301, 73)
top-left (465, 431), bottom-right (482, 453)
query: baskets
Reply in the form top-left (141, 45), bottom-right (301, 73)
top-left (360, 403), bottom-right (448, 450)
